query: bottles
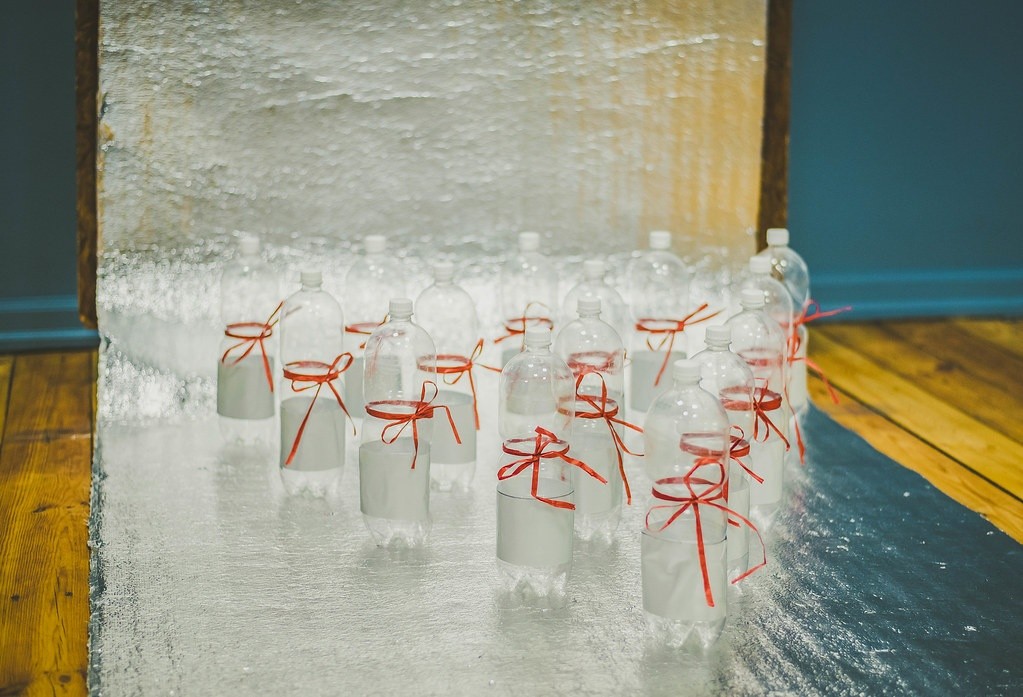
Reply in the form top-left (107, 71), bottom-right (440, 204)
top-left (736, 255), bottom-right (799, 482)
top-left (721, 290), bottom-right (785, 536)
top-left (554, 297), bottom-right (625, 543)
top-left (280, 268), bottom-right (347, 496)
top-left (640, 359), bottom-right (729, 651)
top-left (563, 259), bottom-right (625, 330)
top-left (632, 230), bottom-right (689, 416)
top-left (357, 300), bottom-right (438, 548)
top-left (558, 297), bottom-right (625, 464)
top-left (691, 325), bottom-right (755, 590)
top-left (498, 326), bottom-right (572, 593)
top-left (218, 234), bottom-right (275, 418)
top-left (345, 234), bottom-right (407, 416)
top-left (743, 228), bottom-right (809, 423)
top-left (414, 262), bottom-right (479, 491)
top-left (498, 231), bottom-right (559, 436)
